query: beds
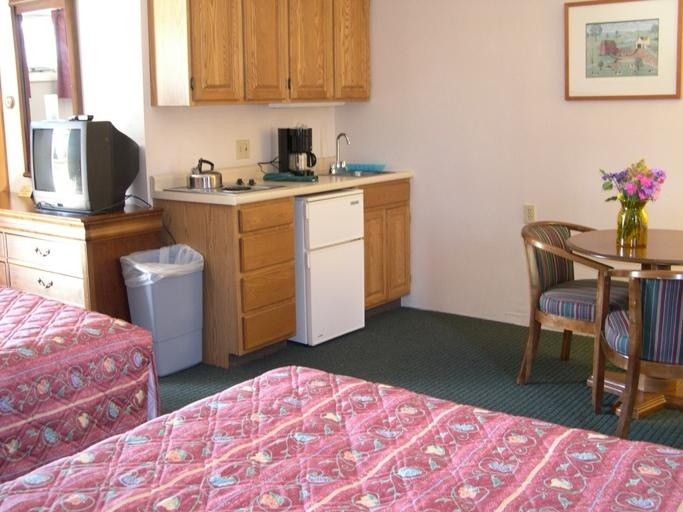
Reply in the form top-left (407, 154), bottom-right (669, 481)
top-left (0, 285), bottom-right (156, 482)
top-left (0, 365), bottom-right (683, 512)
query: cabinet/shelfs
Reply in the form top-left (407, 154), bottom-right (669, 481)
top-left (148, 0), bottom-right (368, 109)
top-left (0, 194), bottom-right (161, 328)
top-left (153, 197), bottom-right (298, 368)
top-left (357, 177), bottom-right (409, 312)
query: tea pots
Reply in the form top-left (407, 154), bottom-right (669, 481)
top-left (188, 158), bottom-right (222, 189)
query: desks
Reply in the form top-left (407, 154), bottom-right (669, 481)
top-left (565, 228), bottom-right (683, 419)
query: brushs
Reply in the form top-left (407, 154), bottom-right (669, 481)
top-left (346, 163), bottom-right (385, 172)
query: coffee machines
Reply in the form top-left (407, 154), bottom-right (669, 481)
top-left (278, 128), bottom-right (317, 176)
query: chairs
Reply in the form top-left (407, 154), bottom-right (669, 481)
top-left (516, 220), bottom-right (644, 416)
top-left (592, 269), bottom-right (683, 441)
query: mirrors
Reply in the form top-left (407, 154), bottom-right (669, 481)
top-left (8, 0), bottom-right (84, 178)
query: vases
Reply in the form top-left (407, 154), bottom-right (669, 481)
top-left (618, 199), bottom-right (649, 249)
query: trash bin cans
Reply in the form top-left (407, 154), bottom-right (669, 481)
top-left (120, 244), bottom-right (204, 377)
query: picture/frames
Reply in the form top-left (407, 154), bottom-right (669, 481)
top-left (563, 1), bottom-right (683, 102)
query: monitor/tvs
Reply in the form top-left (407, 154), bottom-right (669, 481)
top-left (29, 120), bottom-right (140, 214)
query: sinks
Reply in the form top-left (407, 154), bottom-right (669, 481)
top-left (337, 168), bottom-right (396, 179)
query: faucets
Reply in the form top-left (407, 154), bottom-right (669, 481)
top-left (330, 131), bottom-right (352, 176)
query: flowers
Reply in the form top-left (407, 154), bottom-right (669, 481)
top-left (599, 157), bottom-right (667, 201)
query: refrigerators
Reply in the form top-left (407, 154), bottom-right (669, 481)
top-left (287, 190), bottom-right (366, 346)
